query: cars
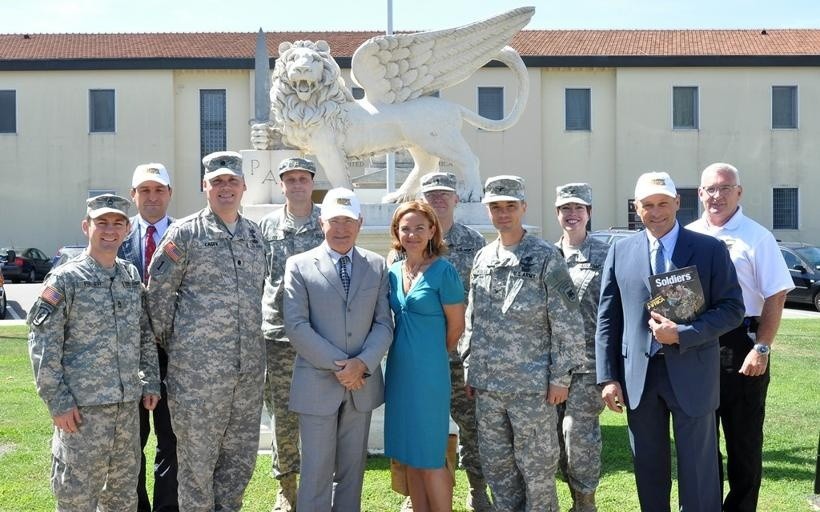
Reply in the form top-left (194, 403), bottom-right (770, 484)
top-left (769, 238), bottom-right (820, 313)
top-left (0, 247), bottom-right (52, 283)
top-left (0, 248), bottom-right (16, 321)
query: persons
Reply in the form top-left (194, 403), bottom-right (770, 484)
top-left (147, 150), bottom-right (272, 511)
top-left (27, 193), bottom-right (162, 512)
top-left (283, 187), bottom-right (394, 511)
top-left (549, 183), bottom-right (607, 510)
top-left (593, 172), bottom-right (744, 512)
top-left (681, 161), bottom-right (796, 511)
top-left (259, 158), bottom-right (363, 510)
top-left (388, 199), bottom-right (466, 512)
top-left (418, 172), bottom-right (493, 512)
top-left (467, 175), bottom-right (585, 511)
top-left (118, 162), bottom-right (178, 512)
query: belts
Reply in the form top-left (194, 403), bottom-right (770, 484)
top-left (656, 346), bottom-right (667, 355)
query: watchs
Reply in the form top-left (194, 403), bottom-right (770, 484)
top-left (751, 342), bottom-right (772, 357)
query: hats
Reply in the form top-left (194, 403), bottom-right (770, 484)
top-left (278, 156), bottom-right (316, 178)
top-left (86, 192), bottom-right (132, 222)
top-left (131, 163), bottom-right (171, 189)
top-left (554, 182), bottom-right (594, 207)
top-left (320, 187), bottom-right (362, 221)
top-left (635, 172), bottom-right (678, 202)
top-left (481, 175), bottom-right (527, 204)
top-left (420, 171), bottom-right (458, 193)
top-left (202, 150), bottom-right (245, 181)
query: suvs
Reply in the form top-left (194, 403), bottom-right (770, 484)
top-left (55, 245), bottom-right (86, 265)
top-left (586, 227), bottom-right (642, 246)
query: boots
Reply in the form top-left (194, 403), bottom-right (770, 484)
top-left (401, 495), bottom-right (412, 512)
top-left (466, 475), bottom-right (494, 511)
top-left (574, 490), bottom-right (598, 512)
top-left (273, 473), bottom-right (298, 512)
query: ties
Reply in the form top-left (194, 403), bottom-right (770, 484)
top-left (651, 243), bottom-right (667, 358)
top-left (145, 224), bottom-right (156, 282)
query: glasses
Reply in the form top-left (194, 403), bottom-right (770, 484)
top-left (698, 184), bottom-right (741, 193)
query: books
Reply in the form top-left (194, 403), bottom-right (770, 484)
top-left (643, 265), bottom-right (706, 325)
